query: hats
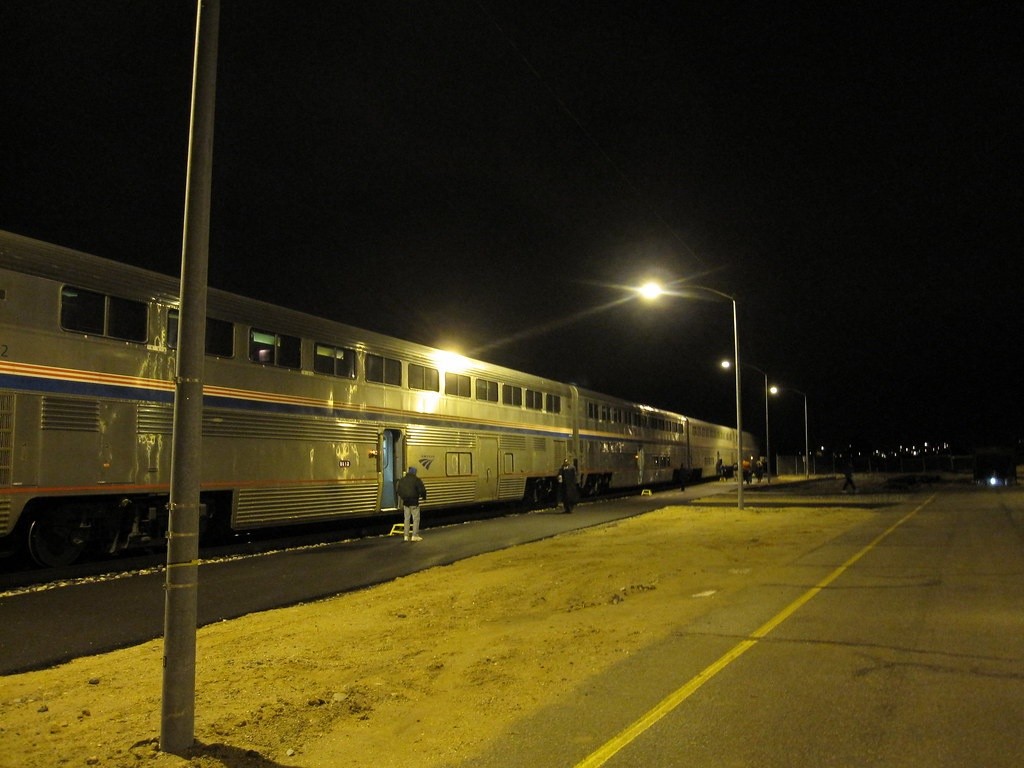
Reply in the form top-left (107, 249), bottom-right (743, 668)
top-left (409, 467), bottom-right (416, 473)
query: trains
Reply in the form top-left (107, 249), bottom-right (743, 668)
top-left (0, 231), bottom-right (761, 591)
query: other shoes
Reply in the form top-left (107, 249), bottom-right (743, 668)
top-left (411, 535), bottom-right (423, 541)
top-left (404, 536), bottom-right (408, 541)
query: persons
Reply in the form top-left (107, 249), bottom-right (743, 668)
top-left (841, 458), bottom-right (859, 493)
top-left (716, 458), bottom-right (723, 481)
top-left (557, 459), bottom-right (578, 513)
top-left (733, 458), bottom-right (765, 484)
top-left (398, 467), bottom-right (426, 541)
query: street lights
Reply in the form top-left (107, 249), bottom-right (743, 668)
top-left (643, 280), bottom-right (744, 510)
top-left (720, 358), bottom-right (771, 485)
top-left (771, 386), bottom-right (809, 479)
